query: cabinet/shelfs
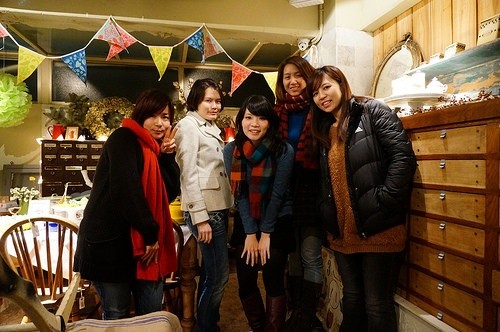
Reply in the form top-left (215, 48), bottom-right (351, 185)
top-left (393, 97), bottom-right (500, 332)
top-left (41, 139), bottom-right (104, 197)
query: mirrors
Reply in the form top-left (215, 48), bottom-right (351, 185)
top-left (365, 32), bottom-right (423, 100)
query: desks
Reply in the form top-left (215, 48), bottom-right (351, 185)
top-left (168, 224), bottom-right (201, 332)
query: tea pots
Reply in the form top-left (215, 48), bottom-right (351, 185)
top-left (427, 77), bottom-right (447, 93)
top-left (169, 199), bottom-right (185, 224)
top-left (47, 123), bottom-right (66, 140)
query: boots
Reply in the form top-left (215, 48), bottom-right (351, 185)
top-left (286, 272), bottom-right (322, 332)
top-left (266, 296), bottom-right (286, 332)
top-left (239, 287), bottom-right (266, 332)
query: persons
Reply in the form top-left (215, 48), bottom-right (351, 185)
top-left (308, 65), bottom-right (418, 332)
top-left (273, 56), bottom-right (329, 332)
top-left (223, 95), bottom-right (296, 332)
top-left (171, 77), bottom-right (236, 332)
top-left (73, 89), bottom-right (180, 321)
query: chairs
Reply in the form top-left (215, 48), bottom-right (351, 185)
top-left (0, 217), bottom-right (103, 332)
top-left (162, 218), bottom-right (184, 318)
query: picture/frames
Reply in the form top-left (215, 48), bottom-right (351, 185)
top-left (64, 126), bottom-right (79, 140)
